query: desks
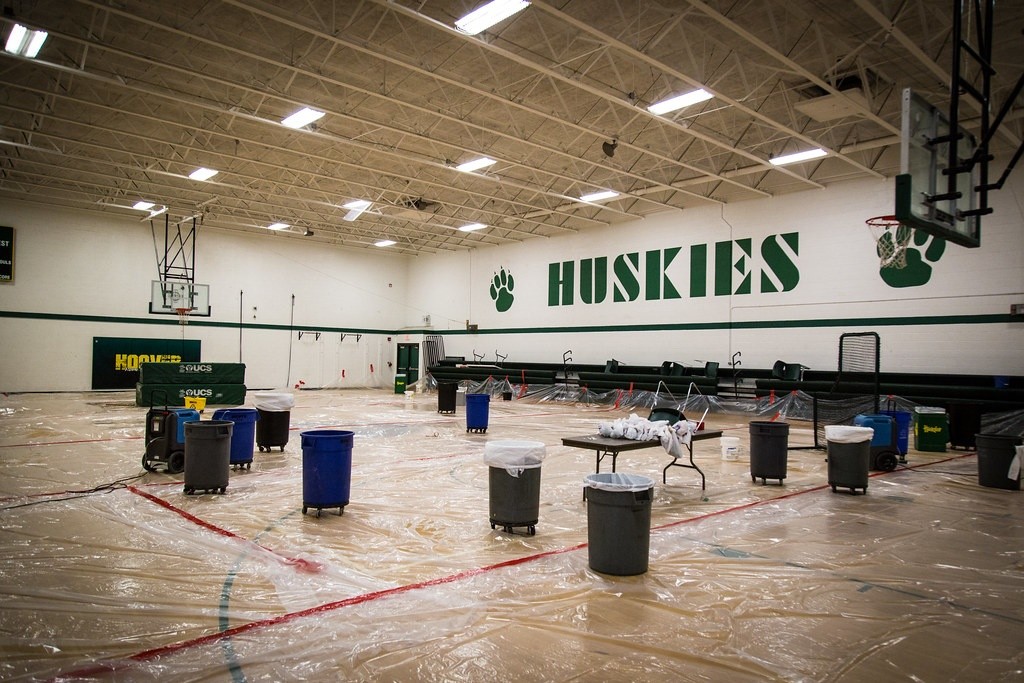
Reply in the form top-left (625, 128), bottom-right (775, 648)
top-left (561, 429), bottom-right (724, 502)
top-left (456, 349), bottom-right (508, 369)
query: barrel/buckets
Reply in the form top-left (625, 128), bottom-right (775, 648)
top-left (503, 391), bottom-right (513, 401)
top-left (395, 374), bottom-right (406, 394)
top-left (720, 436), bottom-right (741, 461)
top-left (437, 381), bottom-right (458, 414)
top-left (466, 393), bottom-right (490, 434)
top-left (485, 439), bottom-right (546, 536)
top-left (583, 472), bottom-right (655, 576)
top-left (184, 392), bottom-right (295, 497)
top-left (749, 399), bottom-right (980, 495)
top-left (300, 429), bottom-right (355, 516)
top-left (975, 433), bottom-right (1024, 490)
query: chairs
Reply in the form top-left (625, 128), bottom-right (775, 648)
top-left (605, 359), bottom-right (625, 374)
top-left (661, 361), bottom-right (684, 377)
top-left (704, 362), bottom-right (719, 377)
top-left (647, 381), bottom-right (710, 434)
top-left (772, 360), bottom-right (810, 381)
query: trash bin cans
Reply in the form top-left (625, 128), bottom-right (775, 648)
top-left (212, 409), bottom-right (260, 472)
top-left (436, 383), bottom-right (459, 414)
top-left (184, 421), bottom-right (235, 494)
top-left (749, 421), bottom-right (790, 487)
top-left (975, 433), bottom-right (1024, 491)
top-left (823, 425), bottom-right (875, 496)
top-left (912, 411), bottom-right (946, 452)
top-left (582, 473), bottom-right (656, 576)
top-left (465, 393), bottom-right (491, 434)
top-left (394, 374), bottom-right (407, 394)
top-left (878, 410), bottom-right (912, 464)
top-left (485, 439), bottom-right (546, 536)
top-left (254, 392), bottom-right (294, 453)
top-left (301, 429), bottom-right (355, 518)
